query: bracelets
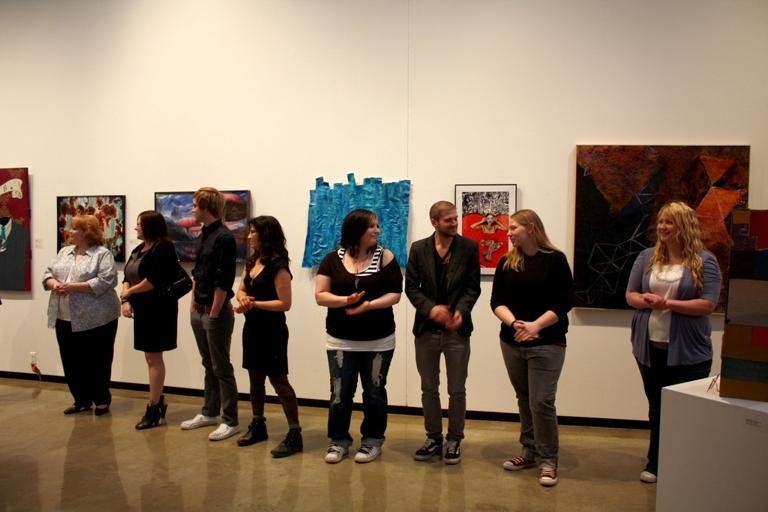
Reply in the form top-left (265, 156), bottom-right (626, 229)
top-left (121, 300), bottom-right (128, 304)
top-left (510, 320), bottom-right (516, 328)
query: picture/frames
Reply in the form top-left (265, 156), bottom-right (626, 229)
top-left (454, 183), bottom-right (517, 277)
top-left (153, 190), bottom-right (252, 265)
top-left (56, 195), bottom-right (126, 263)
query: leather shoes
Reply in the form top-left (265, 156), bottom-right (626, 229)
top-left (95, 401), bottom-right (111, 417)
top-left (62, 401), bottom-right (93, 415)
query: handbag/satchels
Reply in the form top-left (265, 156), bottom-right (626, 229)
top-left (159, 271), bottom-right (192, 299)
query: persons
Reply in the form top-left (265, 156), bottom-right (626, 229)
top-left (120, 210), bottom-right (180, 431)
top-left (179, 186), bottom-right (242, 441)
top-left (231, 216), bottom-right (303, 457)
top-left (41, 212), bottom-right (122, 416)
top-left (489, 210), bottom-right (573, 485)
top-left (313, 210), bottom-right (404, 464)
top-left (406, 200), bottom-right (483, 466)
top-left (625, 200), bottom-right (724, 482)
top-left (470, 212), bottom-right (507, 261)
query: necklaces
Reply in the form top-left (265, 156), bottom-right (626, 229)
top-left (351, 255), bottom-right (369, 273)
top-left (250, 263), bottom-right (263, 286)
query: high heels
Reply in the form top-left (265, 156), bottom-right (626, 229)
top-left (134, 395), bottom-right (168, 430)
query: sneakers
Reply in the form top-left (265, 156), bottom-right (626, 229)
top-left (637, 470), bottom-right (659, 483)
top-left (180, 413), bottom-right (217, 430)
top-left (270, 431), bottom-right (303, 457)
top-left (237, 423), bottom-right (268, 446)
top-left (414, 432), bottom-right (443, 460)
top-left (503, 456), bottom-right (537, 471)
top-left (444, 438), bottom-right (462, 464)
top-left (537, 467), bottom-right (558, 486)
top-left (208, 423), bottom-right (241, 441)
top-left (353, 445), bottom-right (381, 463)
top-left (325, 446), bottom-right (349, 464)
